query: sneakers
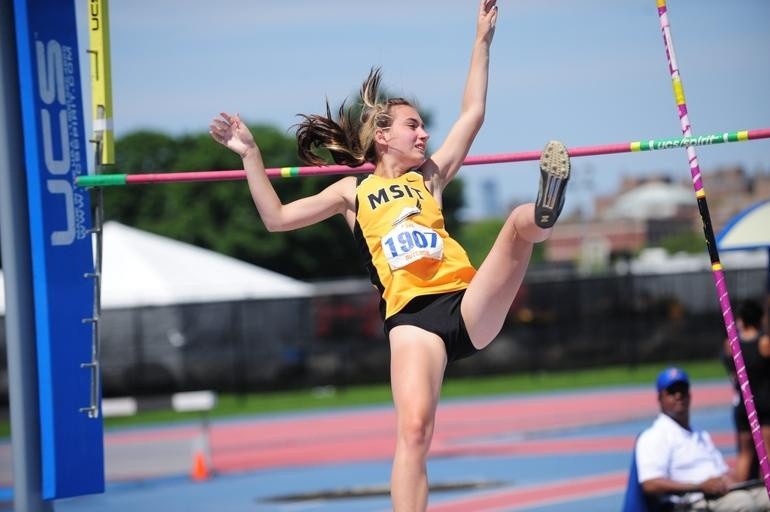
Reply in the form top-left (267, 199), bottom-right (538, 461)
top-left (534, 139), bottom-right (571, 228)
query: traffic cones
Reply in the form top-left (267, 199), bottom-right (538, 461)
top-left (191, 444), bottom-right (207, 483)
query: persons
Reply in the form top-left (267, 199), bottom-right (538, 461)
top-left (634, 296), bottom-right (770, 512)
top-left (209, 0), bottom-right (571, 512)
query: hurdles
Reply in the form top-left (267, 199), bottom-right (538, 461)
top-left (97, 388), bottom-right (217, 476)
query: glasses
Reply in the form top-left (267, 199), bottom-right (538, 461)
top-left (666, 383), bottom-right (688, 393)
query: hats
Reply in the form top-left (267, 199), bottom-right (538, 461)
top-left (656, 367), bottom-right (688, 392)
